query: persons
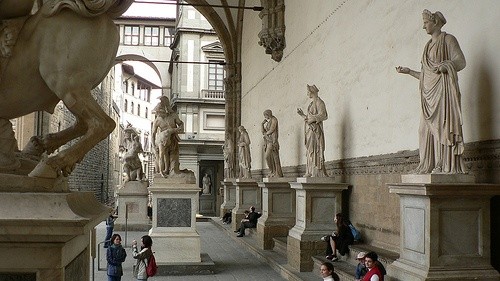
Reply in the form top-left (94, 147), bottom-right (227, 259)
top-left (106, 234), bottom-right (127, 281)
top-left (104, 209), bottom-right (115, 248)
top-left (237, 126), bottom-right (252, 178)
top-left (319, 262), bottom-right (340, 281)
top-left (321, 214), bottom-right (360, 261)
top-left (234, 206), bottom-right (259, 237)
top-left (120, 134), bottom-right (143, 161)
top-left (261, 109), bottom-right (284, 178)
top-left (296, 84), bottom-right (330, 177)
top-left (356, 251), bottom-right (387, 281)
top-left (202, 173), bottom-right (211, 193)
top-left (131, 235), bottom-right (153, 280)
top-left (363, 251), bottom-right (382, 281)
top-left (150, 102), bottom-right (184, 172)
top-left (220, 209), bottom-right (233, 224)
top-left (394, 9), bottom-right (470, 174)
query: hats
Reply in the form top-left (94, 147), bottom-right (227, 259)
top-left (355, 252), bottom-right (367, 260)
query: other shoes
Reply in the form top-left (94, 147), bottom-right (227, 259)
top-left (233, 230), bottom-right (240, 233)
top-left (223, 222), bottom-right (227, 224)
top-left (236, 234), bottom-right (243, 237)
top-left (326, 255), bottom-right (333, 258)
top-left (331, 258), bottom-right (337, 261)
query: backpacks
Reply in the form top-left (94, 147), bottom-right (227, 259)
top-left (142, 252), bottom-right (158, 277)
top-left (349, 224), bottom-right (361, 240)
